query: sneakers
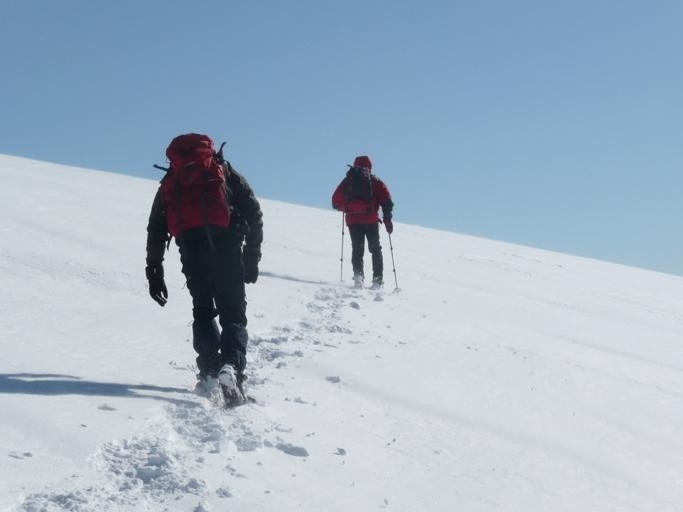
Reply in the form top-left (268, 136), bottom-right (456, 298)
top-left (355, 275), bottom-right (383, 290)
top-left (195, 362), bottom-right (248, 405)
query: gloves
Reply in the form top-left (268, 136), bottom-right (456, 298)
top-left (145, 264), bottom-right (169, 307)
top-left (383, 217), bottom-right (394, 234)
top-left (240, 252), bottom-right (262, 284)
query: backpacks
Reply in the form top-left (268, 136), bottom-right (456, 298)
top-left (160, 133), bottom-right (233, 241)
top-left (344, 168), bottom-right (374, 218)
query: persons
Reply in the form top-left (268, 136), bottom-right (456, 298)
top-left (146, 133), bottom-right (264, 404)
top-left (332, 156), bottom-right (393, 290)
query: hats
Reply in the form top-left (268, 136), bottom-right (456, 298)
top-left (354, 156), bottom-right (373, 169)
